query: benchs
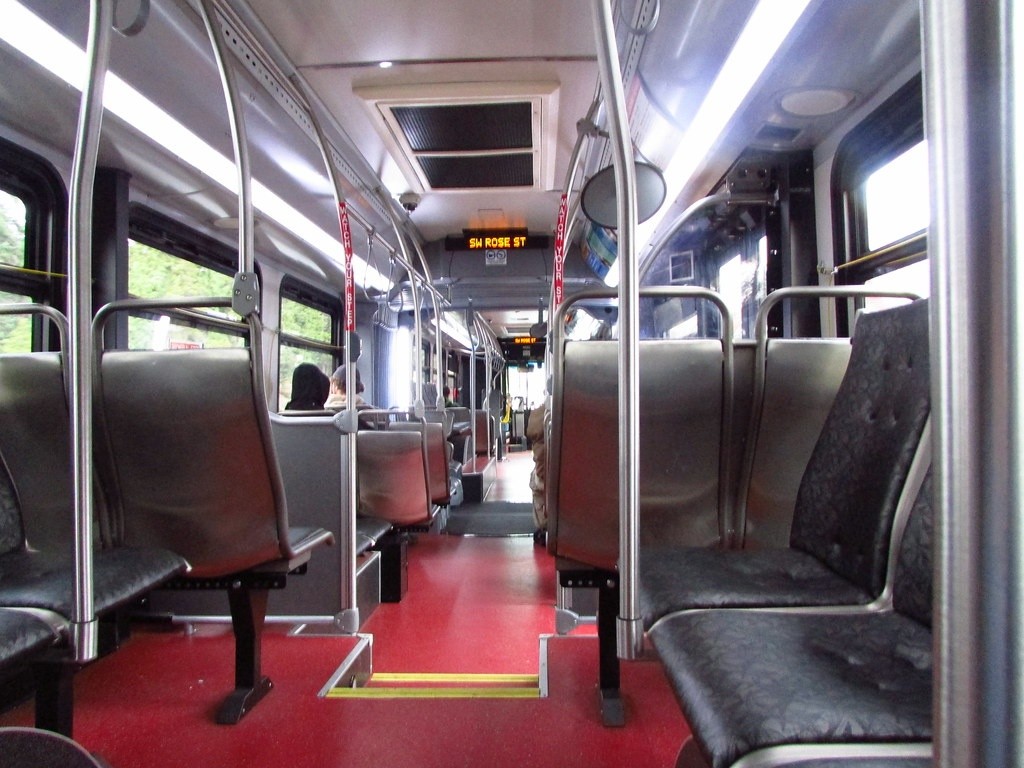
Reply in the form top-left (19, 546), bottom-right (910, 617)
top-left (0, 285), bottom-right (942, 767)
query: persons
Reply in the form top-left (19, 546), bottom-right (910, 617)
top-left (443, 385), bottom-right (459, 408)
top-left (324, 365), bottom-right (376, 430)
top-left (526, 377), bottom-right (551, 543)
top-left (284, 363), bottom-right (330, 416)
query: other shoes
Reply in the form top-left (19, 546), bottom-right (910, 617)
top-left (534, 528), bottom-right (546, 546)
